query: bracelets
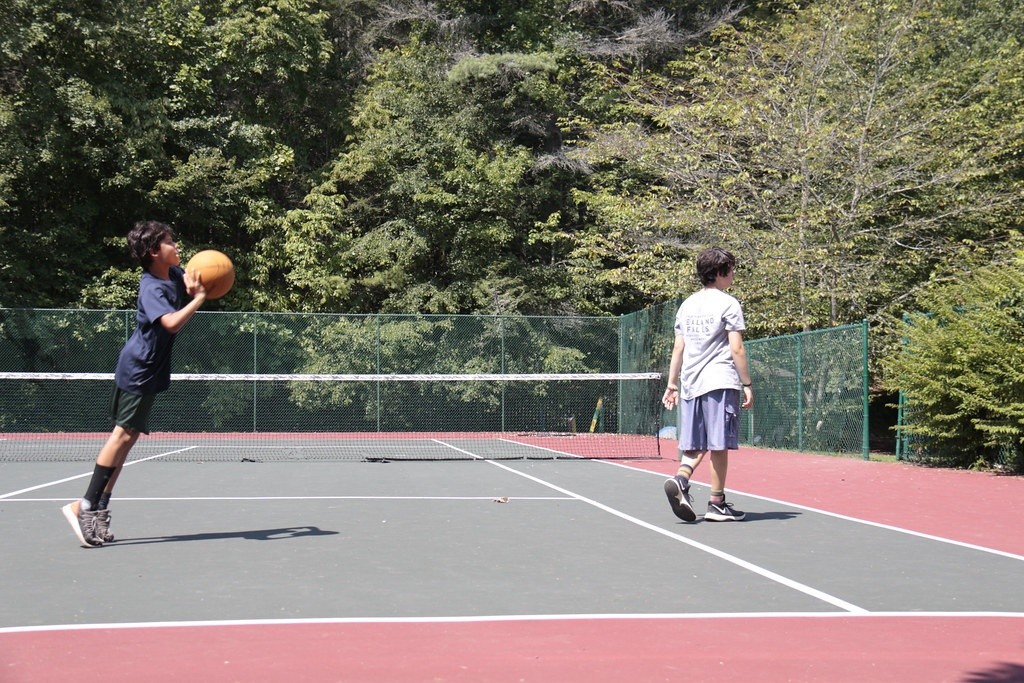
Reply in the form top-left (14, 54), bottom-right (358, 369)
top-left (743, 383), bottom-right (752, 386)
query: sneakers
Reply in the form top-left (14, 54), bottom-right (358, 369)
top-left (62, 501), bottom-right (113, 548)
top-left (664, 475), bottom-right (696, 522)
top-left (704, 494), bottom-right (747, 521)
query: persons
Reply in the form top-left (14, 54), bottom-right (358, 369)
top-left (60, 217), bottom-right (206, 551)
top-left (660, 245), bottom-right (754, 520)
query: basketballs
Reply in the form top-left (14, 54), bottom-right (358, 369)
top-left (184, 250), bottom-right (236, 299)
